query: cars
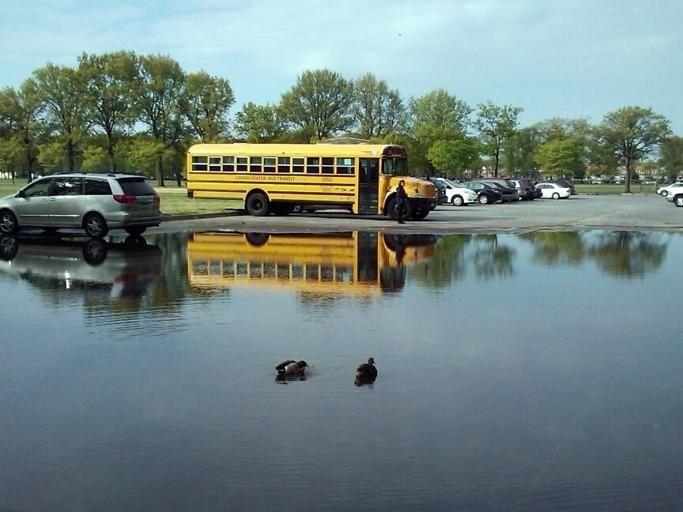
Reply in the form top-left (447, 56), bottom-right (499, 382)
top-left (535, 182), bottom-right (571, 200)
top-left (656, 181), bottom-right (683, 207)
top-left (550, 179), bottom-right (575, 194)
top-left (429, 173), bottom-right (542, 207)
top-left (592, 177), bottom-right (624, 185)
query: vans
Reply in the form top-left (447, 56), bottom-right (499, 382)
top-left (0, 173), bottom-right (164, 237)
top-left (0, 233), bottom-right (162, 301)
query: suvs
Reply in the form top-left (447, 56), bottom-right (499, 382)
top-left (417, 178), bottom-right (448, 211)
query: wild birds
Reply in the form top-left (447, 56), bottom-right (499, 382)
top-left (354, 357), bottom-right (378, 384)
top-left (275, 359), bottom-right (310, 376)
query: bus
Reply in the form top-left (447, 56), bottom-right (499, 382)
top-left (185, 142), bottom-right (437, 219)
top-left (186, 231), bottom-right (435, 296)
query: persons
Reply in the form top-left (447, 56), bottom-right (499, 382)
top-left (395, 180), bottom-right (408, 224)
